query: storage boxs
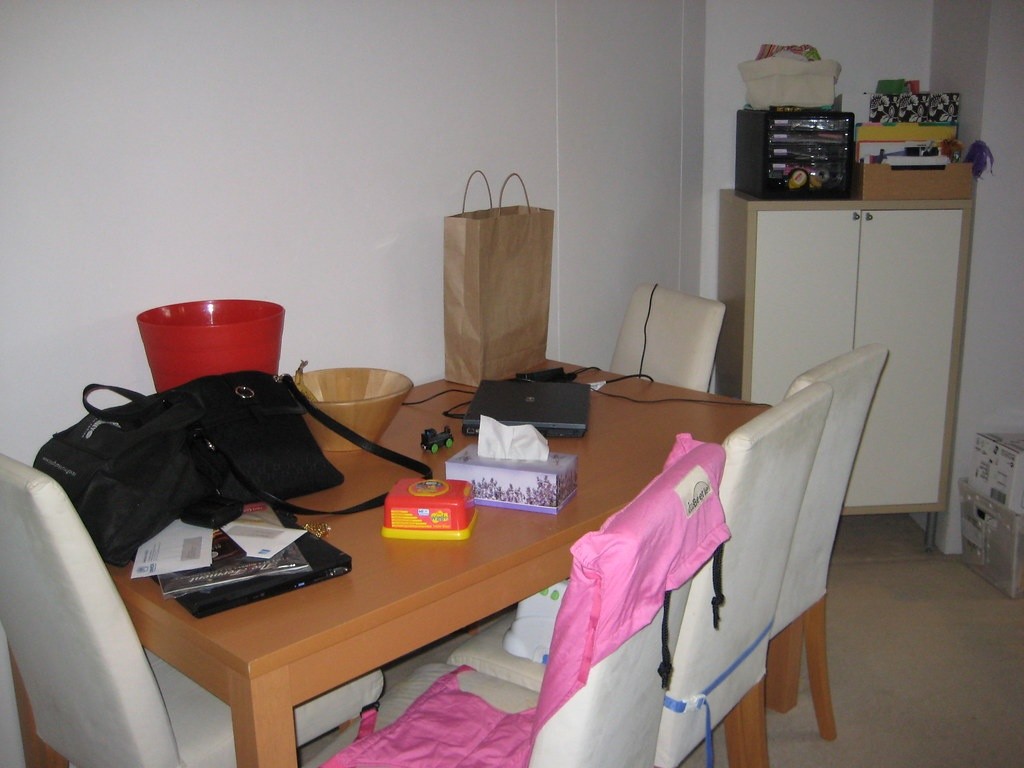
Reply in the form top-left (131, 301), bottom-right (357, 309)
top-left (381, 477), bottom-right (480, 540)
top-left (969, 430), bottom-right (1024, 512)
top-left (735, 108), bottom-right (857, 202)
top-left (957, 477), bottom-right (1024, 600)
top-left (853, 162), bottom-right (975, 203)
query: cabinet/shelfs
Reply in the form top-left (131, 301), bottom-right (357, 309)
top-left (714, 189), bottom-right (975, 554)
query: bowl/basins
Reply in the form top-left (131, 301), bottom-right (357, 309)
top-left (292, 368), bottom-right (414, 451)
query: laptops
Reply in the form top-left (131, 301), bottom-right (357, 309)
top-left (147, 511), bottom-right (351, 619)
top-left (462, 380), bottom-right (591, 438)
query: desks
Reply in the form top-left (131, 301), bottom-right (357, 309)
top-left (9, 357), bottom-right (801, 768)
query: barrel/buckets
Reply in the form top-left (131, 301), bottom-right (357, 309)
top-left (137, 300), bottom-right (286, 393)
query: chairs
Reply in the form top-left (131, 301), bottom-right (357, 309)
top-left (0, 283), bottom-right (892, 768)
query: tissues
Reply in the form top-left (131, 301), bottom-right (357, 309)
top-left (445, 412), bottom-right (578, 515)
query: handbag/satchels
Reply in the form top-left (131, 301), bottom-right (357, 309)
top-left (442, 170), bottom-right (554, 387)
top-left (31, 368), bottom-right (434, 566)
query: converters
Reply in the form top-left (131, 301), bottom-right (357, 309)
top-left (515, 366), bottom-right (565, 383)
